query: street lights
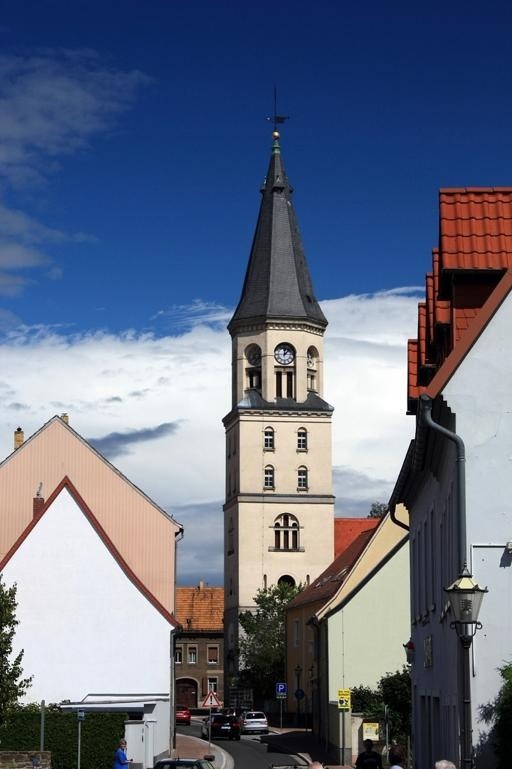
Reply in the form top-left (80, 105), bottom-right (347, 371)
top-left (444, 564), bottom-right (489, 768)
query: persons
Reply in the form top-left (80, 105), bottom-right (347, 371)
top-left (354, 738), bottom-right (384, 769)
top-left (389, 750), bottom-right (404, 769)
top-left (114, 738), bottom-right (133, 769)
top-left (434, 759), bottom-right (456, 769)
top-left (309, 756), bottom-right (324, 769)
top-left (30, 756), bottom-right (39, 769)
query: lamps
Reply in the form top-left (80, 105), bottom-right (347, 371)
top-left (442, 559), bottom-right (490, 651)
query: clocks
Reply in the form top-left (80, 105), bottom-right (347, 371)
top-left (274, 343), bottom-right (296, 366)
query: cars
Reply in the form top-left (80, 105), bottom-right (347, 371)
top-left (240, 711), bottom-right (268, 733)
top-left (175, 704), bottom-right (191, 725)
top-left (147, 749), bottom-right (215, 769)
top-left (201, 713), bottom-right (240, 740)
